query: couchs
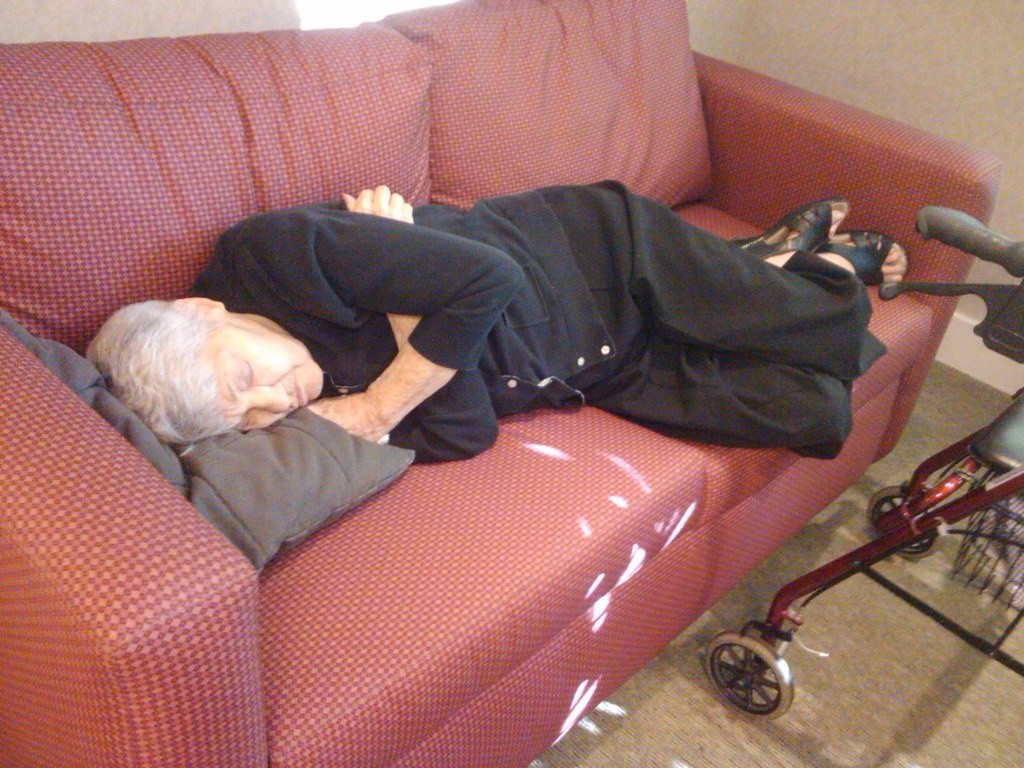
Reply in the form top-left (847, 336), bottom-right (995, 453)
top-left (0, 48), bottom-right (1000, 768)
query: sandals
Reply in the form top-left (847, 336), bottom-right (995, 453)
top-left (812, 230), bottom-right (909, 286)
top-left (728, 197), bottom-right (851, 263)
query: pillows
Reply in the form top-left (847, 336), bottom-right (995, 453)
top-left (374, 0), bottom-right (714, 214)
top-left (0, 305), bottom-right (414, 572)
top-left (0, 18), bottom-right (439, 356)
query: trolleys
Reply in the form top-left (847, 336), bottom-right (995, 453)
top-left (704, 205), bottom-right (1024, 720)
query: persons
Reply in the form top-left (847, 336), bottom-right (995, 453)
top-left (86, 185), bottom-right (907, 465)
